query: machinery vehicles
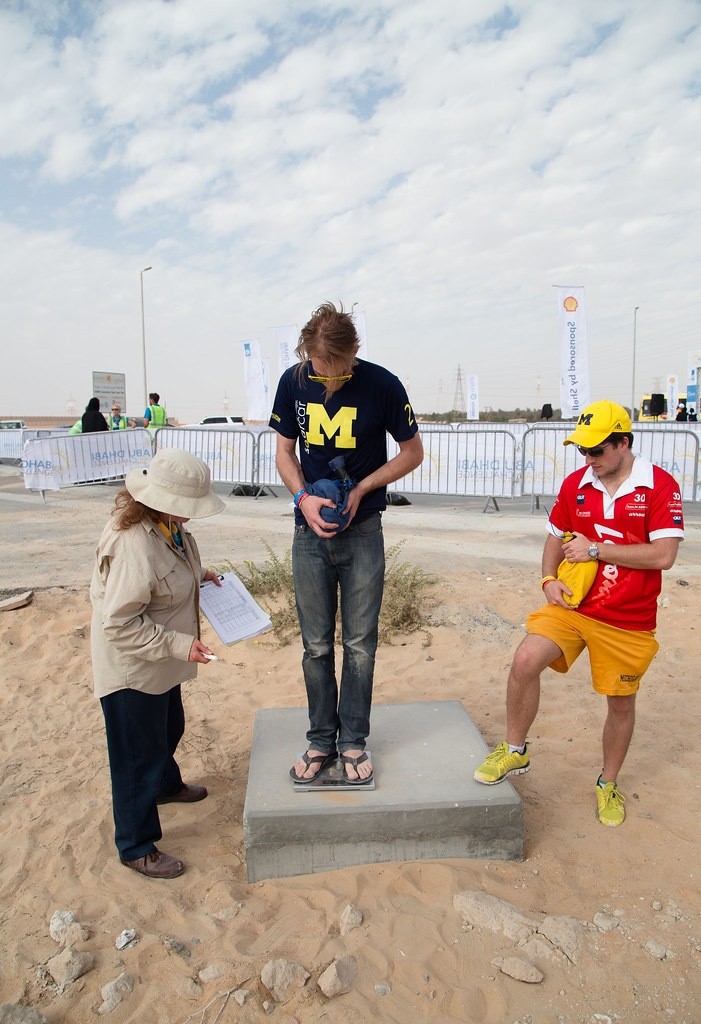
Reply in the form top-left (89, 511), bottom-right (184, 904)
top-left (638, 392), bottom-right (701, 424)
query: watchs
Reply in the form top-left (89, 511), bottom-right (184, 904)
top-left (588, 542), bottom-right (598, 561)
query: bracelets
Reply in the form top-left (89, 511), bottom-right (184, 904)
top-left (540, 575), bottom-right (557, 591)
top-left (294, 490), bottom-right (309, 508)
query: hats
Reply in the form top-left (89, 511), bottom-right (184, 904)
top-left (562, 399), bottom-right (632, 448)
top-left (677, 403), bottom-right (684, 408)
top-left (125, 448), bottom-right (225, 519)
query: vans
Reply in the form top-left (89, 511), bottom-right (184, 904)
top-left (0, 420), bottom-right (26, 430)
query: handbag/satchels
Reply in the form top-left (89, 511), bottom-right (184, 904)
top-left (556, 530), bottom-right (599, 609)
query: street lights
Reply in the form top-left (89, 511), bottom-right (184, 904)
top-left (629, 305), bottom-right (640, 421)
top-left (140, 266), bottom-right (155, 406)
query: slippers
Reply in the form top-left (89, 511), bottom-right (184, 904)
top-left (340, 752), bottom-right (374, 784)
top-left (288, 750), bottom-right (338, 783)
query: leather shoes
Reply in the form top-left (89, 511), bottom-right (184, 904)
top-left (157, 783), bottom-right (208, 804)
top-left (120, 849), bottom-right (186, 879)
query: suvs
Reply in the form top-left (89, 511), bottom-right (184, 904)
top-left (180, 416), bottom-right (246, 427)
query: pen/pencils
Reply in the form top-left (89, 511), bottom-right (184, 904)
top-left (202, 652), bottom-right (219, 661)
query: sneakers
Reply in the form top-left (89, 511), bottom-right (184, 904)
top-left (473, 740), bottom-right (532, 785)
top-left (594, 768), bottom-right (626, 825)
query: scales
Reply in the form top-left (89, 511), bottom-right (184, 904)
top-left (292, 752), bottom-right (375, 792)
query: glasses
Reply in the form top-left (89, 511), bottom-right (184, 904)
top-left (576, 443), bottom-right (612, 457)
top-left (308, 351), bottom-right (352, 382)
top-left (112, 408), bottom-right (118, 411)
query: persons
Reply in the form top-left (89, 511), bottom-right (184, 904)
top-left (267, 299), bottom-right (424, 788)
top-left (91, 448), bottom-right (225, 879)
top-left (473, 400), bottom-right (685, 830)
top-left (674, 404), bottom-right (699, 422)
top-left (67, 393), bottom-right (169, 485)
